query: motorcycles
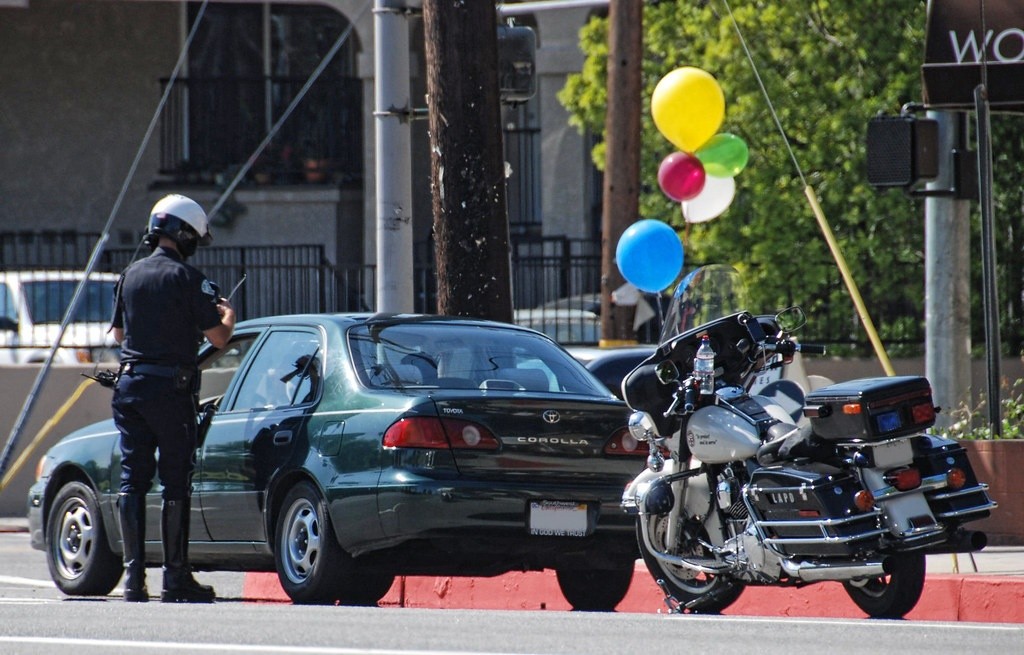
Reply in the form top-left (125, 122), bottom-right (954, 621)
top-left (621, 260), bottom-right (1000, 619)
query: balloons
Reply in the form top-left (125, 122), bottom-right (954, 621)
top-left (615, 219), bottom-right (684, 292)
top-left (651, 67), bottom-right (747, 223)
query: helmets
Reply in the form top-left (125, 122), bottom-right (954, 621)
top-left (148, 193), bottom-right (215, 256)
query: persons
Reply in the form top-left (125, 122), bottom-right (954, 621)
top-left (112, 192), bottom-right (236, 603)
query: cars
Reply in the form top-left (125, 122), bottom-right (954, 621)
top-left (28, 312), bottom-right (672, 606)
top-left (516, 294), bottom-right (663, 399)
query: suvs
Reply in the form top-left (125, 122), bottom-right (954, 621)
top-left (1, 268), bottom-right (121, 366)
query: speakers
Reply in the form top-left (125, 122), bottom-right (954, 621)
top-left (867, 116), bottom-right (940, 187)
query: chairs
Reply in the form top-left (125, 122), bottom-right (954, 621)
top-left (371, 362), bottom-right (425, 384)
top-left (482, 369), bottom-right (549, 391)
top-left (400, 352), bottom-right (438, 381)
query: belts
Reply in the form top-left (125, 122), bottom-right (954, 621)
top-left (120, 363), bottom-right (172, 377)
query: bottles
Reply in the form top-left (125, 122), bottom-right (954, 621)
top-left (696, 335), bottom-right (714, 394)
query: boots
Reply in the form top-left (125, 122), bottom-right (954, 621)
top-left (116, 491), bottom-right (150, 602)
top-left (161, 496), bottom-right (216, 603)
